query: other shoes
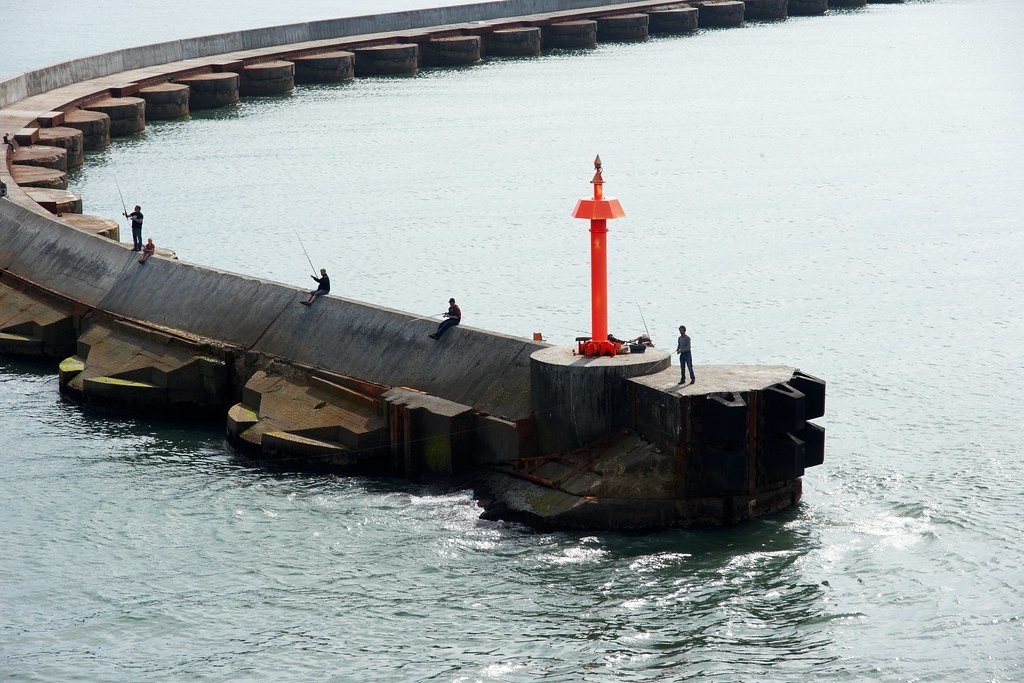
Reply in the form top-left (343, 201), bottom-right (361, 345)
top-left (428, 334), bottom-right (439, 340)
top-left (690, 379), bottom-right (695, 384)
top-left (678, 381), bottom-right (685, 384)
top-left (300, 301), bottom-right (311, 306)
top-left (139, 259), bottom-right (144, 264)
top-left (131, 248), bottom-right (141, 252)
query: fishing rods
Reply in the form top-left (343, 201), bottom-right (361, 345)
top-left (294, 230), bottom-right (318, 279)
top-left (113, 173), bottom-right (129, 220)
top-left (637, 304), bottom-right (651, 341)
top-left (399, 313), bottom-right (444, 325)
top-left (644, 349), bottom-right (679, 375)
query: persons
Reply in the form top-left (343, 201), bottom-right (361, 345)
top-left (676, 326), bottom-right (696, 385)
top-left (138, 238), bottom-right (155, 264)
top-left (3, 133), bottom-right (16, 152)
top-left (300, 269), bottom-right (330, 306)
top-left (428, 298), bottom-right (461, 341)
top-left (127, 205), bottom-right (144, 252)
top-left (631, 334), bottom-right (654, 347)
